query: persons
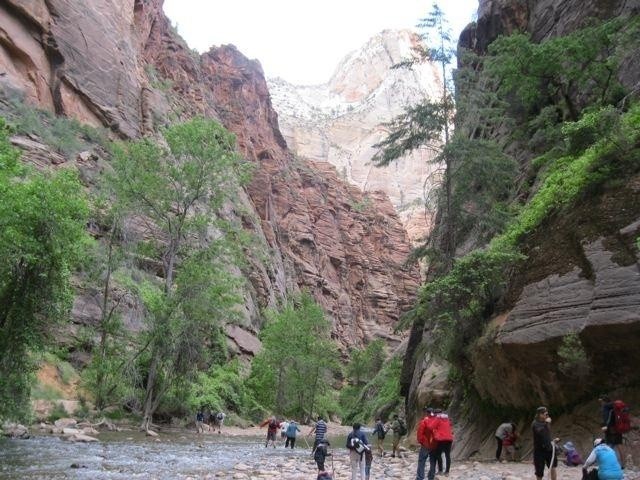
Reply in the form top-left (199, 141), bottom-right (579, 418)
top-left (581, 438), bottom-right (624, 480)
top-left (307, 416), bottom-right (328, 457)
top-left (424, 408), bottom-right (454, 477)
top-left (285, 420), bottom-right (301, 450)
top-left (315, 438), bottom-right (330, 472)
top-left (260, 415), bottom-right (281, 448)
top-left (346, 422), bottom-right (369, 480)
top-left (493, 422), bottom-right (515, 463)
top-left (371, 418), bottom-right (389, 457)
top-left (196, 408), bottom-right (226, 435)
top-left (530, 406), bottom-right (560, 480)
top-left (278, 420), bottom-right (289, 441)
top-left (597, 393), bottom-right (624, 467)
top-left (389, 413), bottom-right (404, 458)
top-left (499, 428), bottom-right (520, 462)
top-left (415, 408), bottom-right (437, 480)
top-left (563, 442), bottom-right (578, 466)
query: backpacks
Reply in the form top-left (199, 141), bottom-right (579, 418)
top-left (581, 468), bottom-right (599, 480)
top-left (314, 448), bottom-right (324, 463)
top-left (397, 419), bottom-right (408, 436)
top-left (350, 437), bottom-right (368, 454)
top-left (218, 413), bottom-right (223, 420)
top-left (612, 399), bottom-right (631, 433)
top-left (380, 423), bottom-right (388, 434)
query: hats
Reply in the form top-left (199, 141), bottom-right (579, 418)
top-left (392, 415), bottom-right (399, 418)
top-left (563, 441), bottom-right (575, 450)
top-left (426, 407), bottom-right (434, 413)
top-left (434, 408), bottom-right (443, 413)
top-left (593, 437), bottom-right (603, 447)
top-left (536, 406), bottom-right (549, 414)
top-left (320, 438), bottom-right (330, 446)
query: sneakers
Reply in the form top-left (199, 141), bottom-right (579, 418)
top-left (381, 450), bottom-right (384, 457)
top-left (436, 470), bottom-right (449, 476)
top-left (390, 454), bottom-right (396, 458)
